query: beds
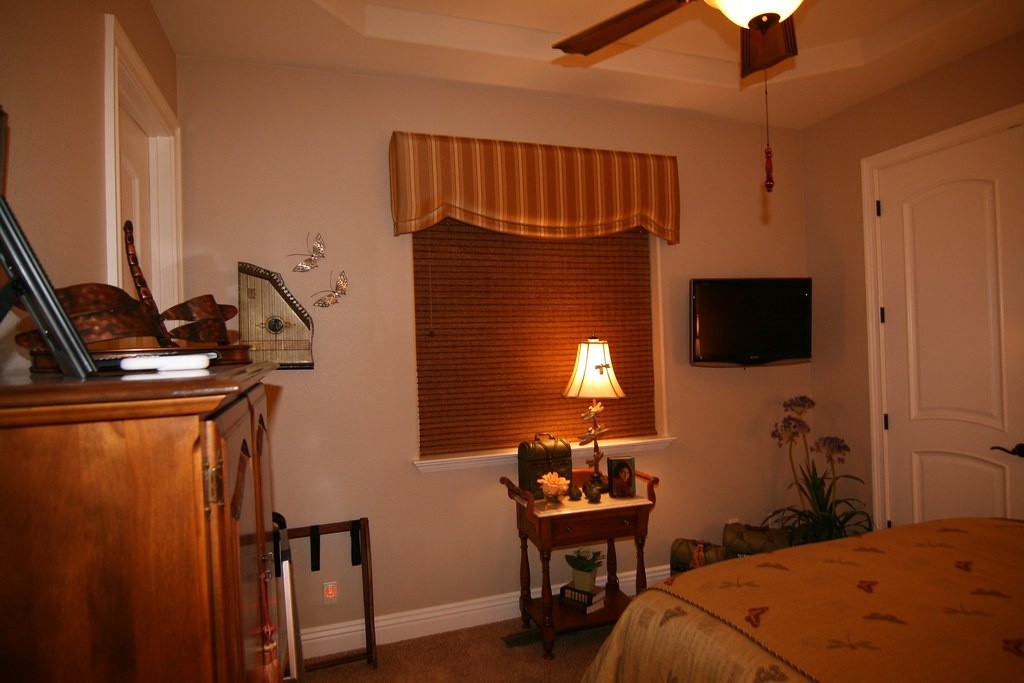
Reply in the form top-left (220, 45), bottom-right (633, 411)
top-left (578, 516), bottom-right (1024, 683)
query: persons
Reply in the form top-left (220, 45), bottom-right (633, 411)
top-left (609, 460), bottom-right (634, 496)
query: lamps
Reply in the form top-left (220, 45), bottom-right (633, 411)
top-left (561, 328), bottom-right (628, 494)
top-left (550, 0), bottom-right (806, 79)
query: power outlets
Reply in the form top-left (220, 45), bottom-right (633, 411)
top-left (322, 581), bottom-right (338, 606)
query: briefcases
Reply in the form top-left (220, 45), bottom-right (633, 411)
top-left (722, 523), bottom-right (791, 556)
top-left (669, 538), bottom-right (728, 574)
top-left (518, 432), bottom-right (573, 500)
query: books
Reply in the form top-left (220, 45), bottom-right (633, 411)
top-left (558, 580), bottom-right (607, 616)
top-left (91, 349), bottom-right (224, 380)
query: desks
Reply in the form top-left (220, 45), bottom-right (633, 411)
top-left (500, 469), bottom-right (660, 661)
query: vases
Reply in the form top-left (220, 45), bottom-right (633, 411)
top-left (543, 494), bottom-right (565, 511)
top-left (573, 567), bottom-right (598, 592)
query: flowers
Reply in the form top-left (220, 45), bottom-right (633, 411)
top-left (759, 394), bottom-right (879, 545)
top-left (565, 547), bottom-right (606, 572)
top-left (537, 471), bottom-right (571, 496)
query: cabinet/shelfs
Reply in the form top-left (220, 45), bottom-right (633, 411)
top-left (0, 361), bottom-right (284, 683)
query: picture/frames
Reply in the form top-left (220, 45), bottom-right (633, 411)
top-left (0, 194), bottom-right (97, 382)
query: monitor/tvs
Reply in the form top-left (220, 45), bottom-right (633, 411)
top-left (688, 277), bottom-right (813, 368)
